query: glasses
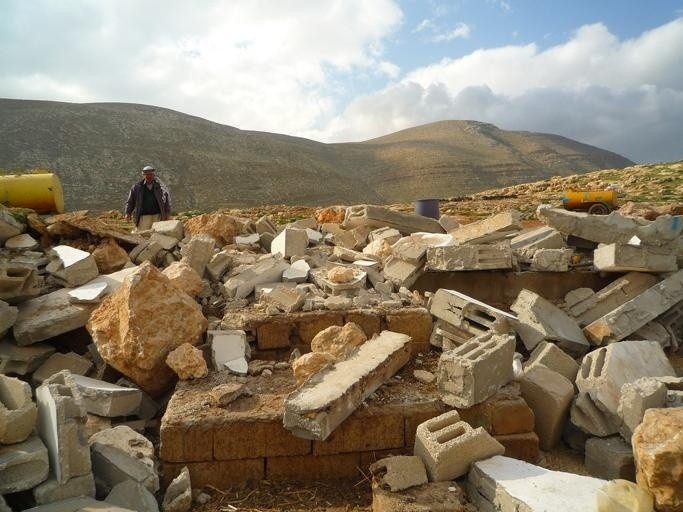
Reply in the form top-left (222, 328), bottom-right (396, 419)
top-left (142, 170), bottom-right (153, 173)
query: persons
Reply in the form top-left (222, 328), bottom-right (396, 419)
top-left (125, 166), bottom-right (171, 232)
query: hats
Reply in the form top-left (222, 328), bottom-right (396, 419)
top-left (142, 166), bottom-right (154, 174)
